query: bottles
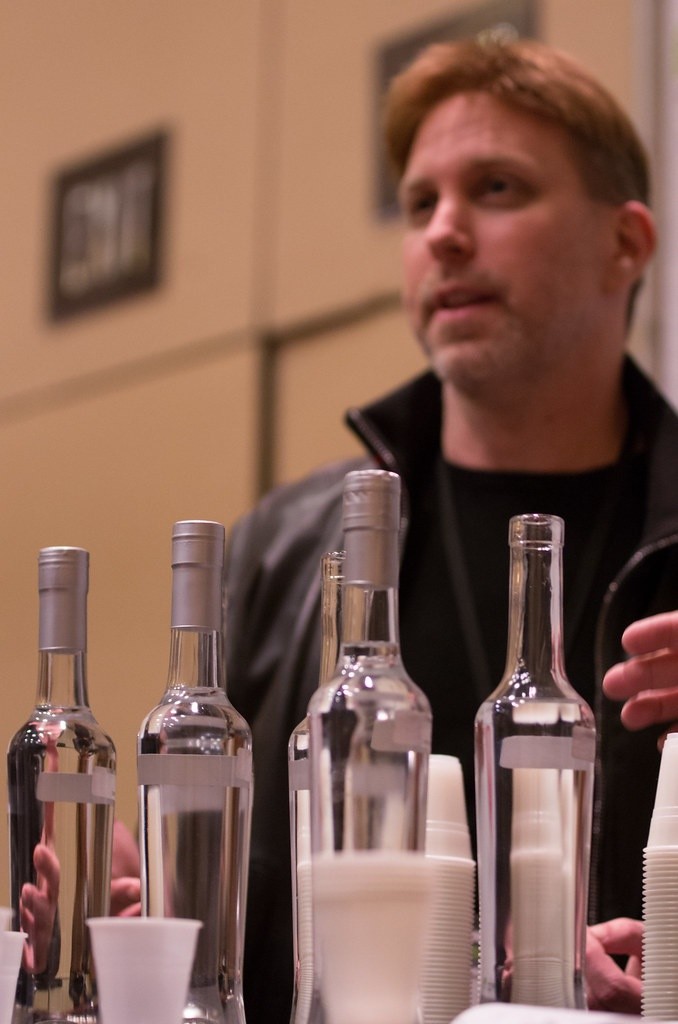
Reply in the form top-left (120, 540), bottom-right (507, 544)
top-left (7, 546), bottom-right (117, 1024)
top-left (306, 469), bottom-right (433, 1024)
top-left (476, 514), bottom-right (594, 1012)
top-left (136, 521), bottom-right (254, 1024)
top-left (287, 550), bottom-right (347, 1024)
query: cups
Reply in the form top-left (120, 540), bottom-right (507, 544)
top-left (1, 907), bottom-right (29, 1024)
top-left (86, 916), bottom-right (204, 1024)
top-left (306, 847), bottom-right (423, 1024)
top-left (423, 755), bottom-right (475, 1024)
top-left (510, 766), bottom-right (577, 1007)
top-left (640, 733), bottom-right (678, 1024)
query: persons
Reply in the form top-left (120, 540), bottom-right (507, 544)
top-left (108, 24), bottom-right (678, 1024)
top-left (8, 721), bottom-right (61, 1005)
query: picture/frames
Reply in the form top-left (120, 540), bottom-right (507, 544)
top-left (369, 0), bottom-right (533, 219)
top-left (47, 133), bottom-right (164, 320)
